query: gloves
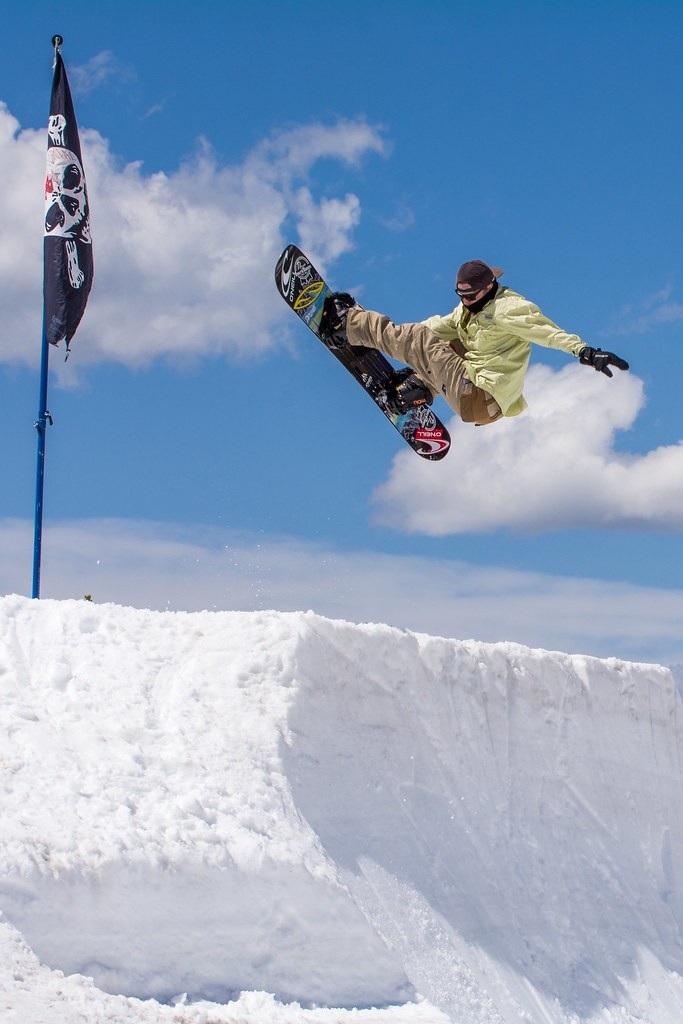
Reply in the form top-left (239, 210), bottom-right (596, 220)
top-left (579, 347), bottom-right (629, 378)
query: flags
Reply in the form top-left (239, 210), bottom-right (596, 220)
top-left (43, 37), bottom-right (93, 363)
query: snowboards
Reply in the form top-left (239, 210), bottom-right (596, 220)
top-left (275, 242), bottom-right (454, 463)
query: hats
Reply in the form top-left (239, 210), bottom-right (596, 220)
top-left (455, 260), bottom-right (503, 290)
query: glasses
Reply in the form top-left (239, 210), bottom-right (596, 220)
top-left (458, 289), bottom-right (481, 301)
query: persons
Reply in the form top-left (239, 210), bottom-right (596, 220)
top-left (325, 258), bottom-right (629, 426)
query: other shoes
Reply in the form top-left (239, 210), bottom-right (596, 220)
top-left (325, 292), bottom-right (357, 349)
top-left (393, 367), bottom-right (417, 411)
